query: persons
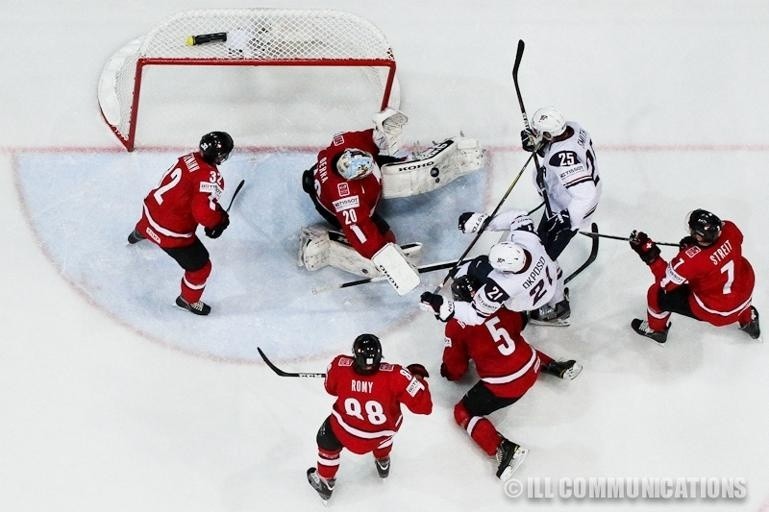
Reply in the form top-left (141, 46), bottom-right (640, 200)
top-left (520, 108), bottom-right (600, 326)
top-left (629, 210), bottom-right (761, 343)
top-left (299, 108), bottom-right (487, 294)
top-left (307, 335), bottom-right (432, 501)
top-left (128, 131), bottom-right (235, 316)
top-left (419, 211), bottom-right (558, 326)
top-left (440, 274), bottom-right (583, 480)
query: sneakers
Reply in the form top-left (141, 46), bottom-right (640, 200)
top-left (738, 305), bottom-right (760, 339)
top-left (307, 467), bottom-right (335, 499)
top-left (530, 287), bottom-right (571, 323)
top-left (127, 231), bottom-right (145, 246)
top-left (631, 319), bottom-right (671, 343)
top-left (495, 439), bottom-right (520, 480)
top-left (175, 296), bottom-right (212, 315)
top-left (546, 359), bottom-right (577, 379)
top-left (375, 457), bottom-right (391, 476)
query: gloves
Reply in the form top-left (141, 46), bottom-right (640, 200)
top-left (539, 208), bottom-right (572, 247)
top-left (628, 230), bottom-right (661, 266)
top-left (371, 106), bottom-right (409, 159)
top-left (406, 363), bottom-right (429, 378)
top-left (520, 129), bottom-right (544, 153)
top-left (371, 242), bottom-right (420, 297)
top-left (204, 209), bottom-right (231, 239)
top-left (416, 290), bottom-right (455, 322)
top-left (458, 212), bottom-right (492, 235)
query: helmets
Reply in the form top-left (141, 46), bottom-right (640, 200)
top-left (688, 209), bottom-right (724, 247)
top-left (529, 108), bottom-right (568, 142)
top-left (450, 275), bottom-right (481, 303)
top-left (488, 241), bottom-right (527, 275)
top-left (334, 146), bottom-right (374, 182)
top-left (199, 130), bottom-right (235, 165)
top-left (350, 333), bottom-right (384, 375)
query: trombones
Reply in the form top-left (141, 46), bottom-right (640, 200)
top-left (255, 346), bottom-right (326, 379)
